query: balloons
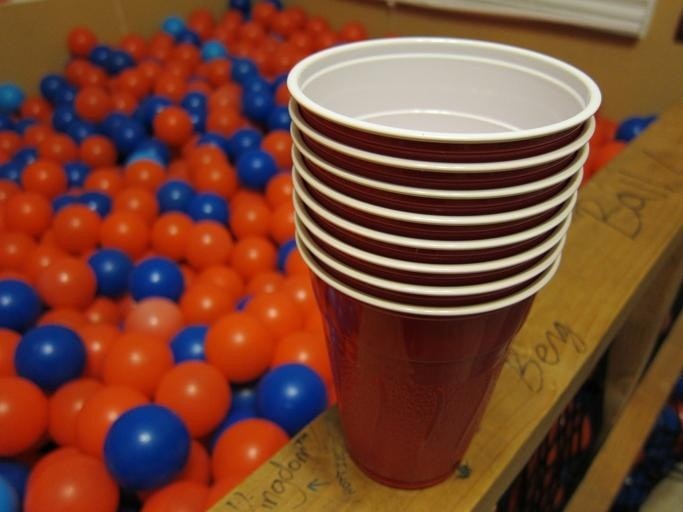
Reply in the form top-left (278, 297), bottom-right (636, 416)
top-left (2, 2), bottom-right (372, 330)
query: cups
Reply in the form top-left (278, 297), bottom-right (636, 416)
top-left (286, 35), bottom-right (604, 492)
